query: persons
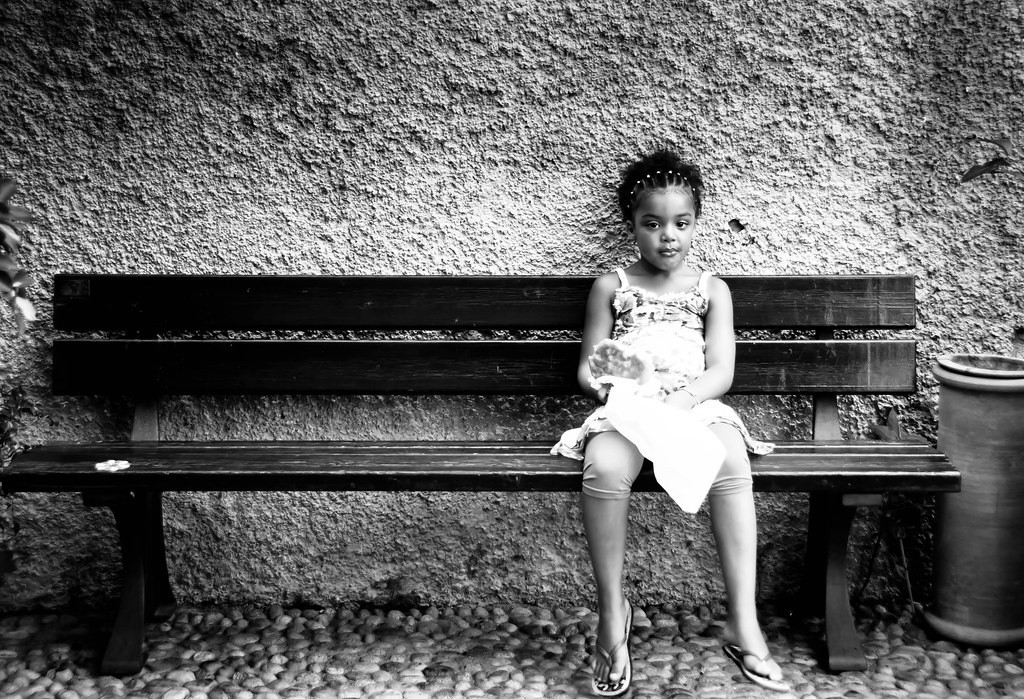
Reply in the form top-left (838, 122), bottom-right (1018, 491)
top-left (580, 149), bottom-right (791, 694)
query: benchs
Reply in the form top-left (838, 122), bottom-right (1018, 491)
top-left (0, 274), bottom-right (963, 678)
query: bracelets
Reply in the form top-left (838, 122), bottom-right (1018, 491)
top-left (679, 387), bottom-right (700, 406)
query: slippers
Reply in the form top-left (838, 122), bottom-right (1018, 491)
top-left (592, 605), bottom-right (632, 695)
top-left (723, 643), bottom-right (790, 692)
